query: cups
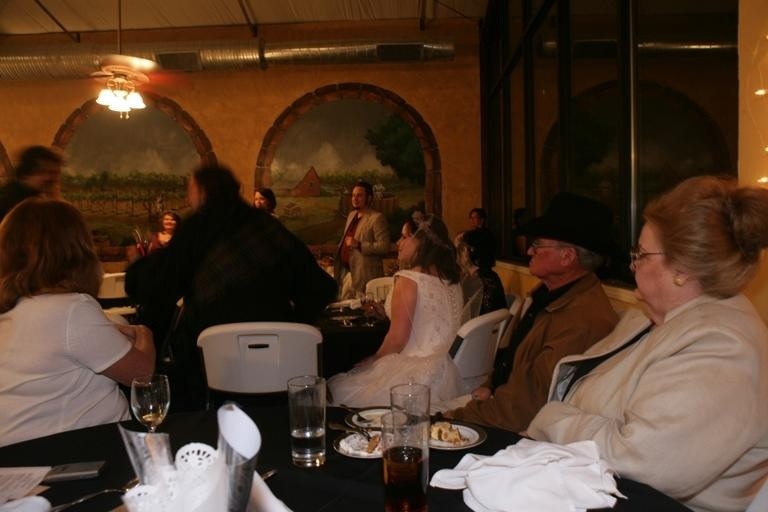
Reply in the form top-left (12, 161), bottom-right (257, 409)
top-left (287, 374), bottom-right (326, 469)
top-left (125, 246), bottom-right (137, 267)
top-left (388, 382), bottom-right (432, 411)
top-left (130, 374), bottom-right (171, 432)
top-left (384, 409), bottom-right (430, 511)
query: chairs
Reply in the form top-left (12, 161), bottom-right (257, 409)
top-left (197, 320), bottom-right (323, 412)
top-left (407, 310), bottom-right (512, 408)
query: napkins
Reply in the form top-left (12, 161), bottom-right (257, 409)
top-left (429, 438), bottom-right (629, 509)
top-left (246, 471), bottom-right (294, 511)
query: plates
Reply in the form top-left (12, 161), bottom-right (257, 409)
top-left (334, 430), bottom-right (393, 459)
top-left (428, 420), bottom-right (488, 450)
top-left (346, 405), bottom-right (413, 432)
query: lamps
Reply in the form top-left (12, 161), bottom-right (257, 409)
top-left (94, 1), bottom-right (154, 121)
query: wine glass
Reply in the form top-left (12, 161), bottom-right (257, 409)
top-left (338, 291), bottom-right (377, 329)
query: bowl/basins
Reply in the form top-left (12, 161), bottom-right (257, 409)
top-left (102, 246), bottom-right (123, 258)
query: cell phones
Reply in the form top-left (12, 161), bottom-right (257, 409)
top-left (44, 457), bottom-right (104, 483)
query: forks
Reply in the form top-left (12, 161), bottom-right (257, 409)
top-left (341, 404), bottom-right (374, 424)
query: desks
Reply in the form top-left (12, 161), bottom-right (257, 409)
top-left (0, 407), bottom-right (690, 511)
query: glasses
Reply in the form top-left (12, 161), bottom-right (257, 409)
top-left (629, 245), bottom-right (670, 269)
top-left (528, 240), bottom-right (577, 253)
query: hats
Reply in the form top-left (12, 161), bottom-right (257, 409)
top-left (513, 190), bottom-right (613, 255)
